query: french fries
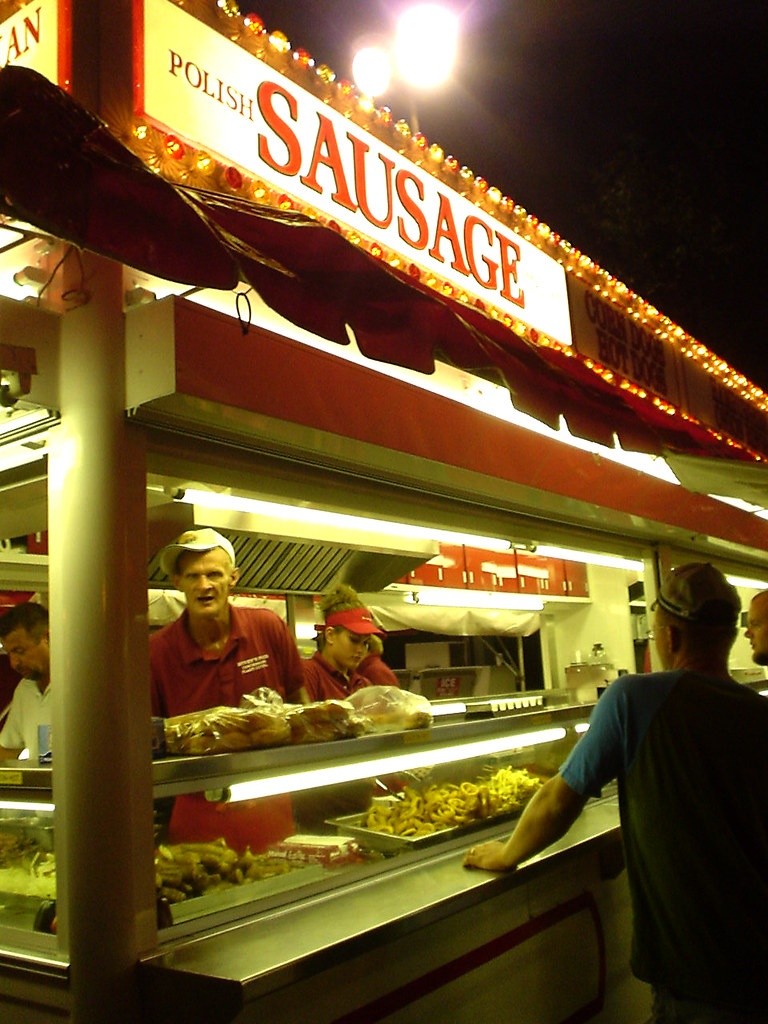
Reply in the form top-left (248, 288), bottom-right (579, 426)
top-left (485, 764), bottom-right (544, 815)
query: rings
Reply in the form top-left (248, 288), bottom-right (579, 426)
top-left (471, 848), bottom-right (475, 854)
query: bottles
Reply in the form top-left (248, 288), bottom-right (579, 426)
top-left (588, 641), bottom-right (616, 702)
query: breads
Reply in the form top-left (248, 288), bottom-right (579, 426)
top-left (155, 837), bottom-right (259, 901)
top-left (162, 684), bottom-right (432, 756)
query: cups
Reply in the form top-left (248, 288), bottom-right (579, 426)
top-left (564, 660), bottom-right (590, 706)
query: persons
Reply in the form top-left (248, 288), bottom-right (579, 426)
top-left (744, 590), bottom-right (768, 666)
top-left (146, 528), bottom-right (310, 857)
top-left (291, 582), bottom-right (409, 836)
top-left (0, 603), bottom-right (52, 764)
top-left (462, 563), bottom-right (768, 1024)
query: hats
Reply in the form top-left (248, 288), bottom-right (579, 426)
top-left (160, 528), bottom-right (234, 575)
top-left (314, 607), bottom-right (387, 639)
top-left (651, 561), bottom-right (741, 624)
